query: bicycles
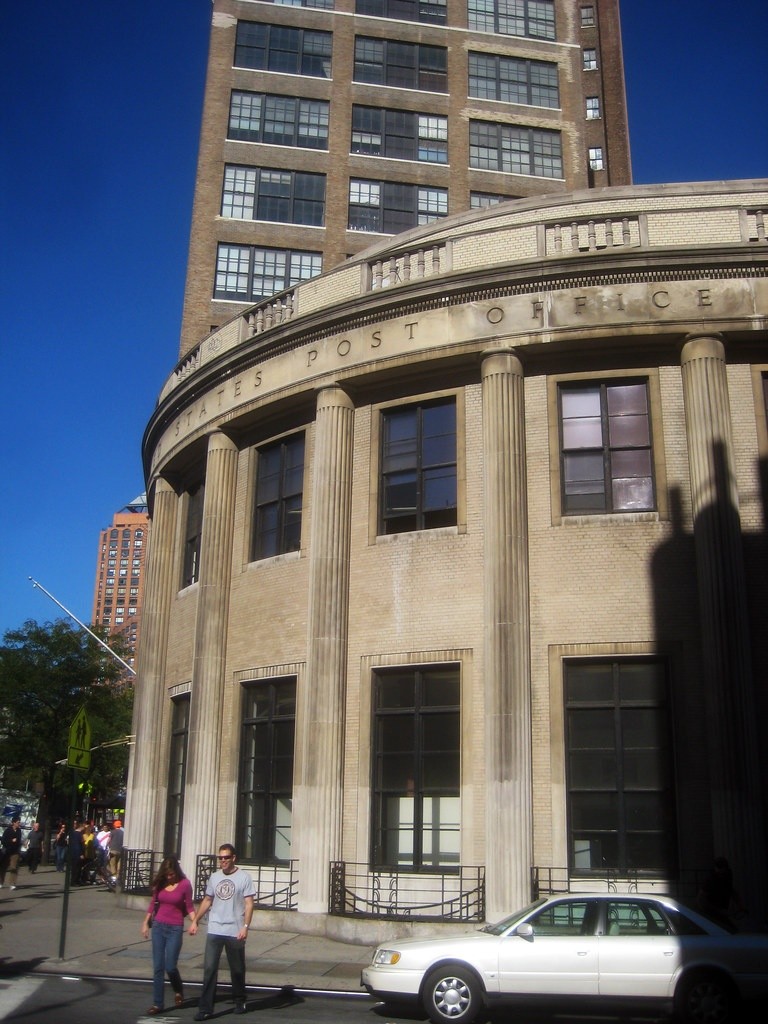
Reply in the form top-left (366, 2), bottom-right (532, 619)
top-left (81, 857), bottom-right (115, 892)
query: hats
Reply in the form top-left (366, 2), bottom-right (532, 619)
top-left (114, 821), bottom-right (122, 828)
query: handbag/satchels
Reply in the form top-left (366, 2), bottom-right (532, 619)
top-left (148, 891), bottom-right (159, 928)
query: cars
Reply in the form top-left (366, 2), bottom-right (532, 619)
top-left (360, 892), bottom-right (768, 1024)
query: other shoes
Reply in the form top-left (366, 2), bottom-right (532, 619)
top-left (0, 884), bottom-right (2, 888)
top-left (193, 1011), bottom-right (211, 1021)
top-left (175, 991), bottom-right (183, 1005)
top-left (147, 1005), bottom-right (161, 1014)
top-left (234, 1001), bottom-right (246, 1014)
top-left (10, 886), bottom-right (16, 890)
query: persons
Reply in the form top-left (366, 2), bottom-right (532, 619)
top-left (55, 820), bottom-right (124, 887)
top-left (0, 815), bottom-right (21, 891)
top-left (188, 843), bottom-right (254, 1021)
top-left (24, 823), bottom-right (44, 874)
top-left (141, 855), bottom-right (198, 1015)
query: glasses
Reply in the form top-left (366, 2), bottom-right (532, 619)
top-left (218, 854), bottom-right (233, 860)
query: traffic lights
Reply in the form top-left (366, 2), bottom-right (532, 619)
top-left (91, 795), bottom-right (98, 802)
top-left (76, 779), bottom-right (88, 797)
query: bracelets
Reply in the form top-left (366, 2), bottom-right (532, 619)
top-left (245, 923), bottom-right (248, 928)
